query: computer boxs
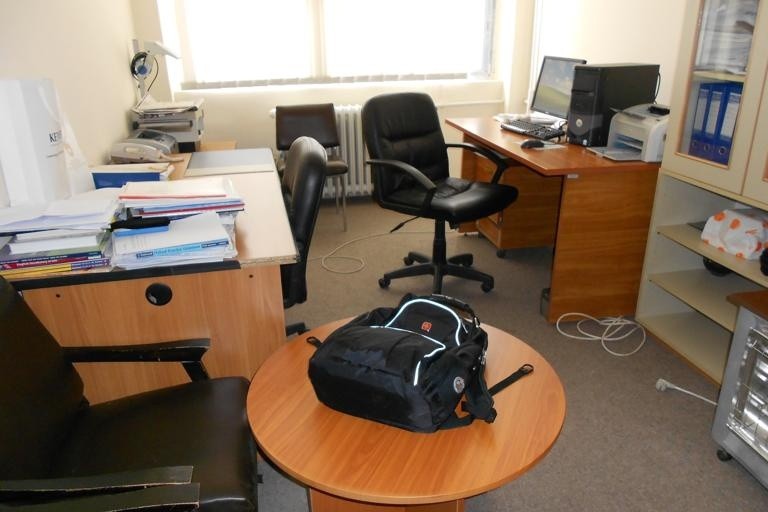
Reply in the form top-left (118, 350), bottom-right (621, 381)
top-left (566, 64), bottom-right (660, 146)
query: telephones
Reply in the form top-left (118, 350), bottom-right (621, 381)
top-left (110, 141), bottom-right (186, 162)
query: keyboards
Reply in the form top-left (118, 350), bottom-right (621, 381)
top-left (500, 119), bottom-right (566, 141)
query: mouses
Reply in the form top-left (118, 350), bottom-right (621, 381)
top-left (521, 140), bottom-right (545, 148)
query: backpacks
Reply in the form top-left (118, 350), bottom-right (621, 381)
top-left (304, 290), bottom-right (535, 434)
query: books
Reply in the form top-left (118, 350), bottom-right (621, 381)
top-left (118, 180), bottom-right (246, 224)
top-left (112, 209), bottom-right (230, 260)
top-left (0, 214), bottom-right (113, 279)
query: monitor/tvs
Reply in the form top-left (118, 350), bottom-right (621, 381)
top-left (530, 56), bottom-right (587, 129)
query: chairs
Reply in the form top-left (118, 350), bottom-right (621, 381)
top-left (0, 275), bottom-right (258, 512)
top-left (276, 103), bottom-right (349, 231)
top-left (280, 136), bottom-right (328, 335)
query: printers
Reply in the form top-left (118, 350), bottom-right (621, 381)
top-left (586, 103), bottom-right (671, 163)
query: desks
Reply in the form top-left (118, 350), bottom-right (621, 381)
top-left (198, 141), bottom-right (236, 151)
top-left (247, 314), bottom-right (566, 512)
top-left (6, 147), bottom-right (300, 407)
top-left (445, 116), bottom-right (662, 324)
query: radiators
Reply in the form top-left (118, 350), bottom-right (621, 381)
top-left (269, 103), bottom-right (374, 199)
top-left (361, 91), bottom-right (519, 295)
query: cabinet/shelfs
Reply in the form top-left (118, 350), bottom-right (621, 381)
top-left (661, 0), bottom-right (768, 205)
top-left (633, 167), bottom-right (767, 390)
